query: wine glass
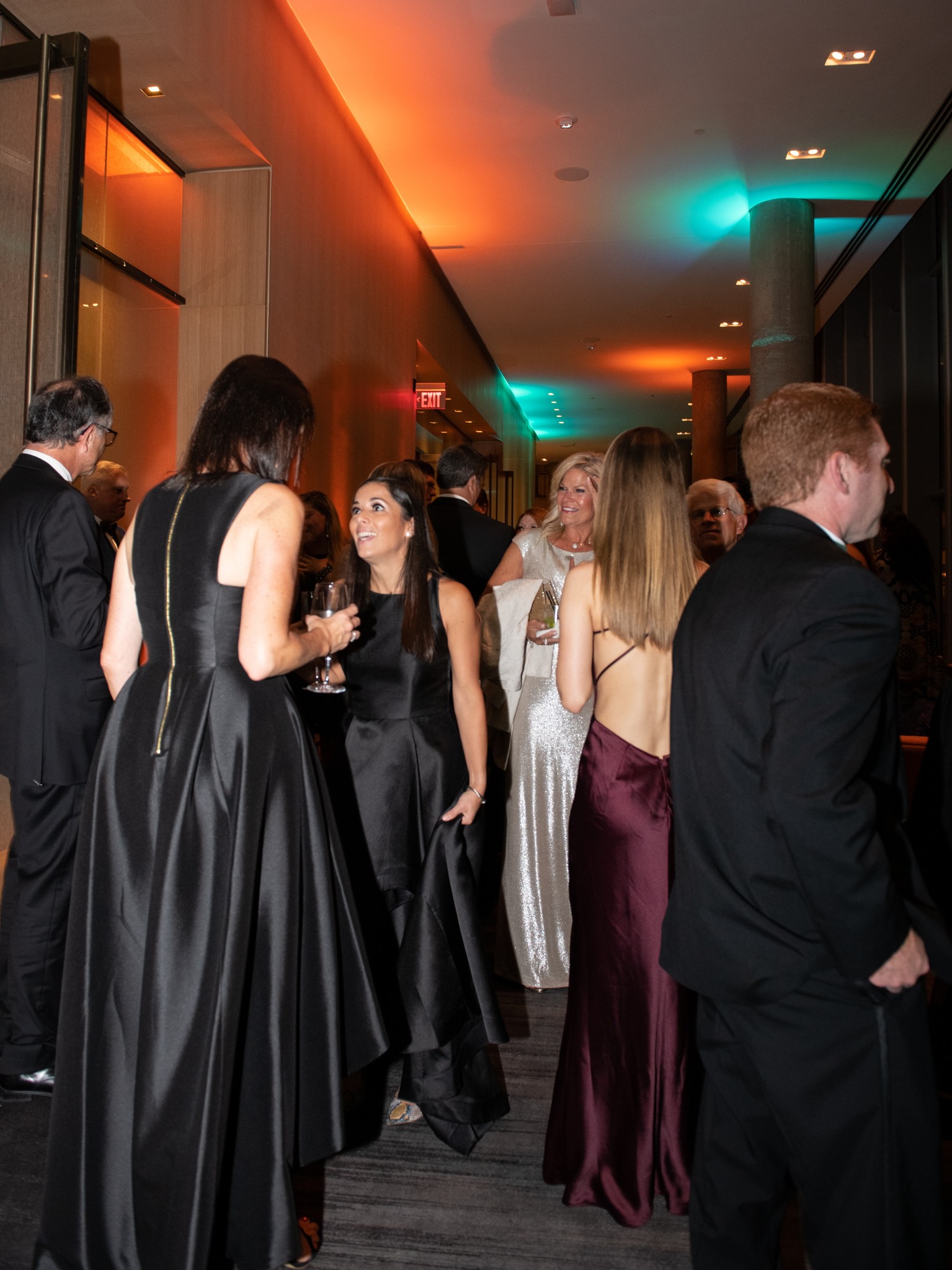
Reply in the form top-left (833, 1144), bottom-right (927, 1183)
top-left (299, 589), bottom-right (328, 690)
top-left (307, 581), bottom-right (350, 693)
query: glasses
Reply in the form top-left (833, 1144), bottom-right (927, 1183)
top-left (688, 506), bottom-right (738, 520)
top-left (80, 423), bottom-right (118, 446)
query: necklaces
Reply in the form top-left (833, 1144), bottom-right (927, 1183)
top-left (562, 532), bottom-right (594, 548)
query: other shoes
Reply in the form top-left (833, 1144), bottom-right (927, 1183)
top-left (386, 1097), bottom-right (422, 1126)
top-left (284, 1211), bottom-right (324, 1269)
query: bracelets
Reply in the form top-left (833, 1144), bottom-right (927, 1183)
top-left (467, 786), bottom-right (486, 803)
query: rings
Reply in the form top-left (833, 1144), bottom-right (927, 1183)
top-left (350, 631), bottom-right (356, 643)
top-left (544, 639), bottom-right (548, 645)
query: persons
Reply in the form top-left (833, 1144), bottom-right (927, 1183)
top-left (0, 355), bottom-right (951, 1269)
